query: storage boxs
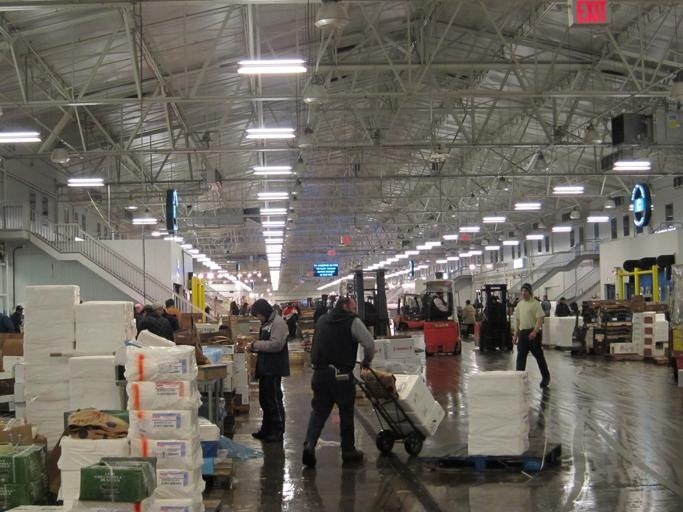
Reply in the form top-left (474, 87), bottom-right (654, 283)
top-left (78, 455), bottom-right (158, 504)
top-left (0, 415), bottom-right (49, 512)
top-left (353, 337), bottom-right (422, 387)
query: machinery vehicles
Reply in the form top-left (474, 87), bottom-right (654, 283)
top-left (298, 290), bottom-right (346, 338)
top-left (421, 279), bottom-right (462, 355)
top-left (344, 268), bottom-right (425, 355)
top-left (465, 282), bottom-right (514, 351)
top-left (391, 291), bottom-right (424, 331)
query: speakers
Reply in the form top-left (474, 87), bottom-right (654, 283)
top-left (612, 113), bottom-right (646, 144)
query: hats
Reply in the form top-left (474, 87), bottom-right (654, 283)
top-left (521, 284), bottom-right (531, 291)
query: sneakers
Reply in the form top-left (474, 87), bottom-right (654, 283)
top-left (341, 447), bottom-right (364, 463)
top-left (303, 451), bottom-right (316, 467)
top-left (252, 431), bottom-right (284, 443)
top-left (540, 374), bottom-right (550, 387)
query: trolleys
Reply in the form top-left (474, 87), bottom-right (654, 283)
top-left (351, 357), bottom-right (425, 453)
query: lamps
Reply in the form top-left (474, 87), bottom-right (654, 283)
top-left (235, 54), bottom-right (308, 201)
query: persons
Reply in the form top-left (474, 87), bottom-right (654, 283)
top-left (135, 298), bottom-right (179, 342)
top-left (0, 313), bottom-right (15, 333)
top-left (246, 299), bottom-right (290, 443)
top-left (511, 282), bottom-right (550, 388)
top-left (218, 298), bottom-right (299, 341)
top-left (429, 288), bottom-right (592, 345)
top-left (302, 297), bottom-right (376, 466)
top-left (11, 305), bottom-right (24, 333)
